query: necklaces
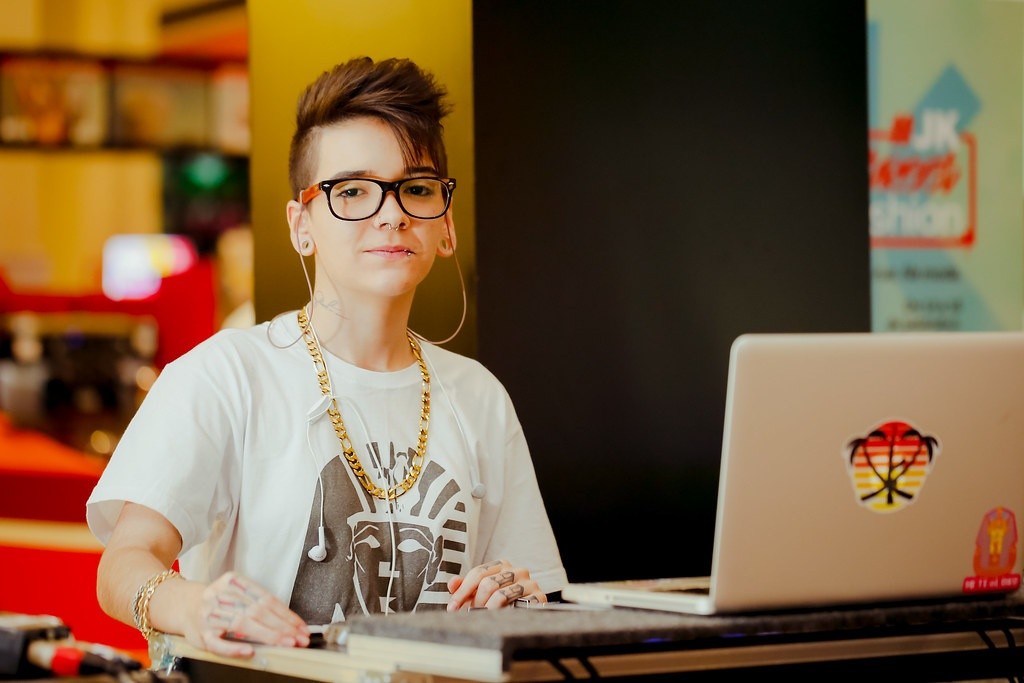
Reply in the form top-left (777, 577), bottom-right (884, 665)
top-left (293, 307), bottom-right (432, 499)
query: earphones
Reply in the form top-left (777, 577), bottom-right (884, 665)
top-left (308, 526), bottom-right (327, 561)
top-left (471, 473), bottom-right (487, 498)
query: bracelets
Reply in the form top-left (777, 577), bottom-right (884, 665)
top-left (131, 569), bottom-right (177, 640)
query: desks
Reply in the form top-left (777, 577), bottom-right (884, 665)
top-left (159, 615), bottom-right (1024, 683)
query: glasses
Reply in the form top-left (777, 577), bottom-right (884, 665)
top-left (297, 176), bottom-right (457, 222)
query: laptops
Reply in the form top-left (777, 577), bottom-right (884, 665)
top-left (560, 331), bottom-right (1024, 617)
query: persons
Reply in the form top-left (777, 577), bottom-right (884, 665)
top-left (85, 55), bottom-right (569, 661)
